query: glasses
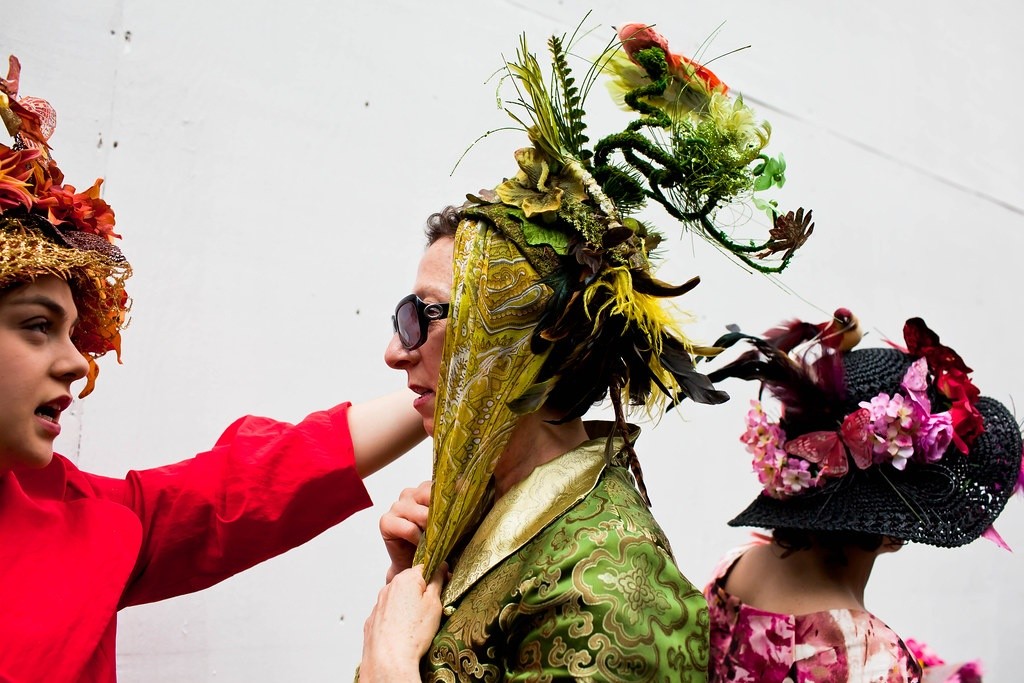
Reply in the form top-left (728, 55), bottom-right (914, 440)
top-left (391, 293), bottom-right (450, 351)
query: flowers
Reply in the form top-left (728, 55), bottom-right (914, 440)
top-left (739, 357), bottom-right (986, 499)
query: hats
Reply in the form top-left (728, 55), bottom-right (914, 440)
top-left (665, 308), bottom-right (1024, 548)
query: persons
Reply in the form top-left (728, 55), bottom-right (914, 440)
top-left (0, 273), bottom-right (428, 683)
top-left (703, 348), bottom-right (1024, 683)
top-left (355, 207), bottom-right (711, 683)
